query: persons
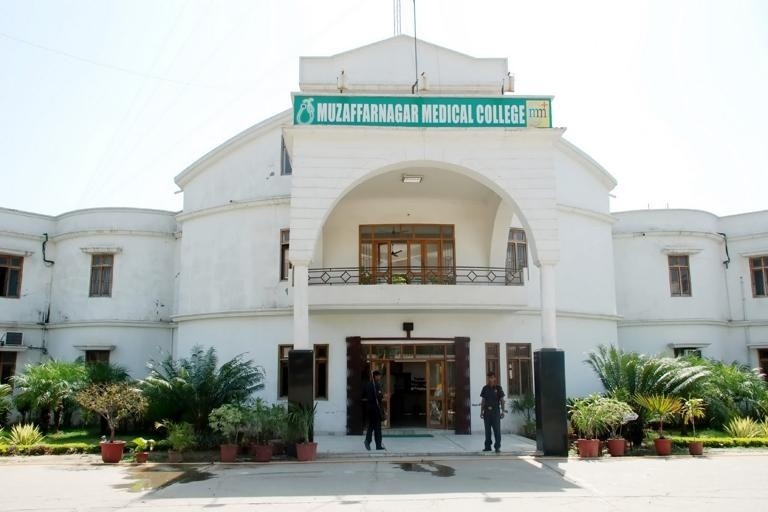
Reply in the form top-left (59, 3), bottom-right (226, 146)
top-left (364, 370), bottom-right (385, 450)
top-left (480, 371), bottom-right (506, 453)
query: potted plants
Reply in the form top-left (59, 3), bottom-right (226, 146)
top-left (566, 392), bottom-right (710, 458)
top-left (72, 378), bottom-right (317, 465)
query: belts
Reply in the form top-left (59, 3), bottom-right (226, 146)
top-left (484, 406), bottom-right (499, 410)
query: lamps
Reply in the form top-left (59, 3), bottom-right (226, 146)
top-left (402, 174), bottom-right (423, 184)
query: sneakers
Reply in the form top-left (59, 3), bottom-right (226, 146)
top-left (483, 446), bottom-right (493, 452)
top-left (364, 439), bottom-right (372, 450)
top-left (495, 448), bottom-right (501, 453)
top-left (376, 446), bottom-right (385, 450)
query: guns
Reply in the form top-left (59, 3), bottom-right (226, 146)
top-left (370, 370), bottom-right (388, 422)
